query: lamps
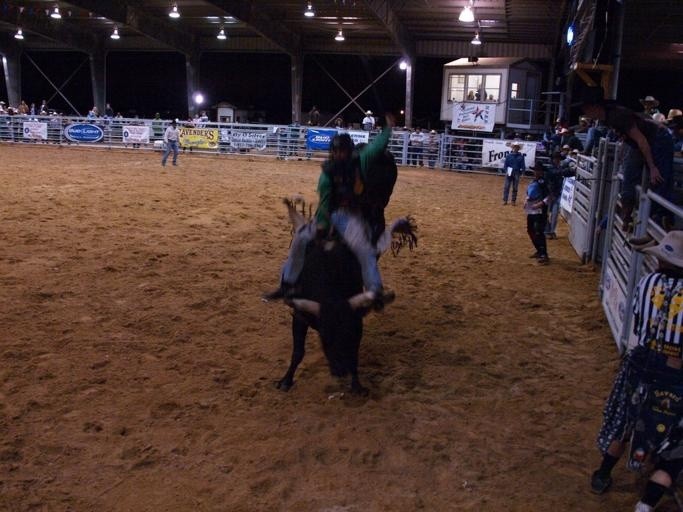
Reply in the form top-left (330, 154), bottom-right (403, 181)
top-left (334, 25), bottom-right (345, 42)
top-left (168, 2), bottom-right (180, 19)
top-left (51, 6), bottom-right (61, 19)
top-left (217, 24), bottom-right (226, 40)
top-left (459, 1), bottom-right (475, 22)
top-left (15, 27), bottom-right (23, 40)
top-left (470, 28), bottom-right (481, 46)
top-left (304, 1), bottom-right (314, 18)
top-left (110, 24), bottom-right (120, 40)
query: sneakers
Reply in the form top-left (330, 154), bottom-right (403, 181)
top-left (540, 256), bottom-right (548, 266)
top-left (589, 471), bottom-right (612, 495)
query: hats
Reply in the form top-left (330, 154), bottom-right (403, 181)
top-left (639, 96), bottom-right (682, 122)
top-left (511, 141), bottom-right (523, 149)
top-left (169, 121), bottom-right (178, 124)
top-left (573, 86), bottom-right (616, 107)
top-left (365, 110), bottom-right (374, 115)
top-left (643, 231), bottom-right (682, 268)
top-left (529, 161), bottom-right (548, 170)
top-left (560, 128), bottom-right (570, 134)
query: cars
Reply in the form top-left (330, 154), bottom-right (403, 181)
top-left (36, 109), bottom-right (185, 141)
top-left (370, 126), bottom-right (463, 154)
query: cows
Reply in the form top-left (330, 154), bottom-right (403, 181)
top-left (273, 215), bottom-right (419, 398)
